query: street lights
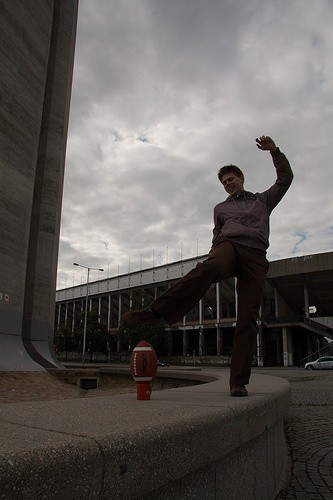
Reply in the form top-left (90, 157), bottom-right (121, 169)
top-left (74, 263), bottom-right (104, 365)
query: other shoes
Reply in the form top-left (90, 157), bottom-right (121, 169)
top-left (121, 307), bottom-right (156, 326)
top-left (229, 384), bottom-right (248, 396)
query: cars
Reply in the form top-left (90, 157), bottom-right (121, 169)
top-left (305, 356), bottom-right (333, 369)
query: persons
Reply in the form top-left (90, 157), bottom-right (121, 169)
top-left (121, 135), bottom-right (293, 396)
